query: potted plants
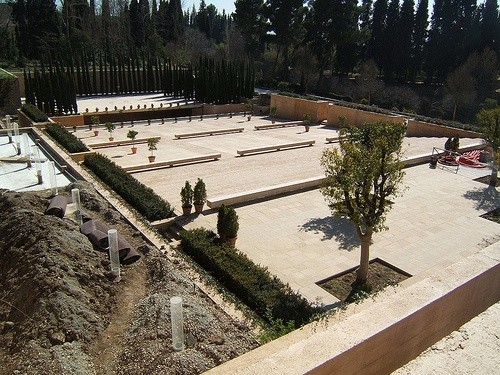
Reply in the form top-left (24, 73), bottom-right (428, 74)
top-left (127, 129), bottom-right (139, 154)
top-left (271, 106), bottom-right (277, 124)
top-left (303, 113), bottom-right (311, 132)
top-left (217, 204), bottom-right (239, 248)
top-left (246, 103), bottom-right (253, 121)
top-left (179, 180), bottom-right (194, 216)
top-left (193, 178), bottom-right (207, 214)
top-left (91, 116), bottom-right (100, 136)
top-left (147, 137), bottom-right (159, 163)
top-left (104, 122), bottom-right (116, 141)
